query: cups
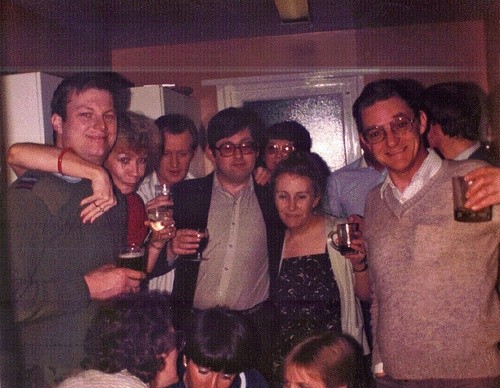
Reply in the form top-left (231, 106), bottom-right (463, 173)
top-left (119, 243), bottom-right (144, 273)
top-left (328, 222), bottom-right (359, 256)
top-left (452, 176), bottom-right (492, 223)
top-left (155, 184), bottom-right (175, 209)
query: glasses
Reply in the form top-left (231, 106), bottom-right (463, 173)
top-left (363, 116), bottom-right (417, 144)
top-left (263, 142), bottom-right (296, 155)
top-left (215, 142), bottom-right (258, 157)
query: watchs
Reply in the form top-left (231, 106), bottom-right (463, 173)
top-left (353, 260), bottom-right (369, 272)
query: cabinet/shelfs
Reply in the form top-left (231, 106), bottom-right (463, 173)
top-left (3, 72), bottom-right (204, 180)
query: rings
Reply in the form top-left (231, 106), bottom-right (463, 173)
top-left (93, 202), bottom-right (99, 207)
top-left (100, 206), bottom-right (104, 212)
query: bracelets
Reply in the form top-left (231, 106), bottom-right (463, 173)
top-left (58, 150), bottom-right (66, 175)
top-left (149, 241), bottom-right (162, 250)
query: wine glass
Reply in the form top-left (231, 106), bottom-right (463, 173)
top-left (190, 228), bottom-right (209, 262)
top-left (147, 208), bottom-right (169, 243)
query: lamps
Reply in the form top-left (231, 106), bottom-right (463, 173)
top-left (273, 0), bottom-right (311, 26)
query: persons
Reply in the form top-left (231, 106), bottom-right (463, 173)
top-left (136, 113), bottom-right (200, 294)
top-left (270, 152), bottom-right (372, 359)
top-left (253, 120), bottom-right (312, 186)
top-left (322, 75), bottom-right (424, 219)
top-left (280, 331), bottom-right (366, 388)
top-left (57, 289), bottom-right (187, 388)
top-left (464, 167), bottom-right (500, 211)
top-left (8, 109), bottom-right (177, 281)
top-left (177, 307), bottom-right (269, 388)
top-left (424, 83), bottom-right (500, 165)
top-left (173, 107), bottom-right (288, 374)
top-left (357, 80), bottom-right (500, 388)
top-left (0, 74), bottom-right (147, 388)
